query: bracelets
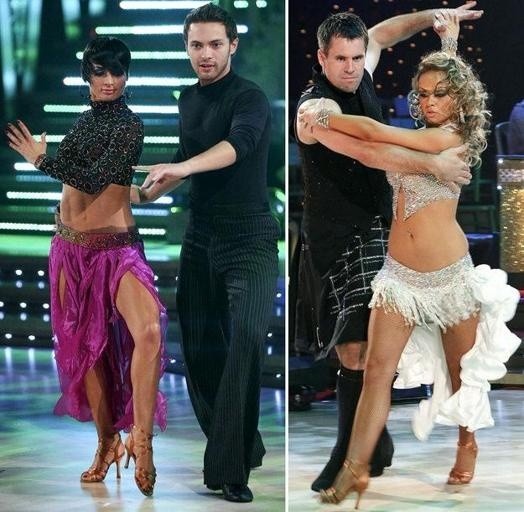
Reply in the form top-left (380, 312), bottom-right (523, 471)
top-left (316, 106), bottom-right (333, 128)
top-left (33, 152), bottom-right (48, 169)
top-left (441, 37), bottom-right (458, 52)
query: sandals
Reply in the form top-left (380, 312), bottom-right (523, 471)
top-left (446, 438), bottom-right (478, 486)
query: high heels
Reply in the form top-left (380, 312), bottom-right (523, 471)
top-left (311, 457), bottom-right (370, 511)
top-left (80, 431), bottom-right (125, 483)
top-left (124, 423), bottom-right (157, 497)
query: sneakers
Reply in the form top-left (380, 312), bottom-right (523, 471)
top-left (312, 431), bottom-right (394, 492)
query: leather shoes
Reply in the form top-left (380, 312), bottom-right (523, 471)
top-left (221, 481), bottom-right (254, 503)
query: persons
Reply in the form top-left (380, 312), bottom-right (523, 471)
top-left (130, 2), bottom-right (279, 501)
top-left (295, 0), bottom-right (487, 490)
top-left (298, 7), bottom-right (522, 509)
top-left (6, 30), bottom-right (169, 495)
